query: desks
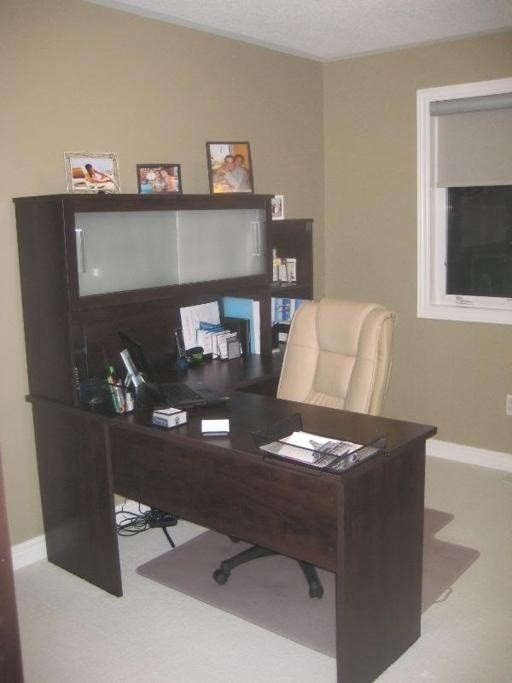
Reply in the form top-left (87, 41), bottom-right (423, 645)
top-left (25, 354), bottom-right (436, 683)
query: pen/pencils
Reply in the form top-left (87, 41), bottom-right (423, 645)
top-left (106, 366), bottom-right (134, 414)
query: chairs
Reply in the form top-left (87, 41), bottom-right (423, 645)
top-left (211, 299), bottom-right (397, 599)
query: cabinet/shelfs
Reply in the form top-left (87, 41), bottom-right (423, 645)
top-left (15, 197), bottom-right (313, 409)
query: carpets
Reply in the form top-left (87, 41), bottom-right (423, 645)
top-left (136, 506), bottom-right (480, 658)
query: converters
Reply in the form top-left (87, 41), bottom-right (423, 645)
top-left (149, 518), bottom-right (176, 529)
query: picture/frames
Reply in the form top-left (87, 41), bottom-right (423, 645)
top-left (63, 141), bottom-right (257, 194)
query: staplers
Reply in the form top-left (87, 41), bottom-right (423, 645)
top-left (184, 347), bottom-right (204, 365)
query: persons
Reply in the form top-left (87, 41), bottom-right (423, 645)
top-left (159, 167), bottom-right (179, 192)
top-left (231, 153), bottom-right (252, 192)
top-left (152, 169), bottom-right (166, 193)
top-left (215, 153), bottom-right (241, 191)
top-left (83, 160), bottom-right (115, 190)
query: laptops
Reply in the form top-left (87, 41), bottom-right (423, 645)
top-left (114, 327), bottom-right (230, 409)
